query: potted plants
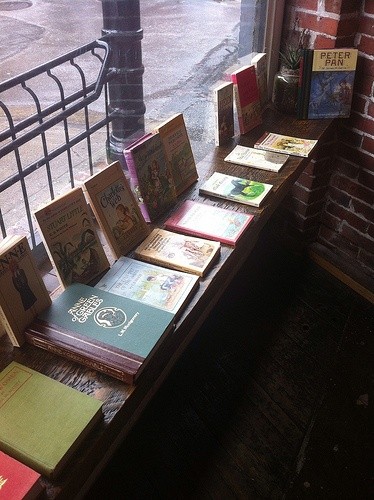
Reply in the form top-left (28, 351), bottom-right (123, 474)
top-left (271, 49), bottom-right (304, 109)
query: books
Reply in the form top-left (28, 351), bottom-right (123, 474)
top-left (0, 48), bottom-right (358, 500)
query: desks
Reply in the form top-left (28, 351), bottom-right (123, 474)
top-left (0, 112), bottom-right (333, 500)
